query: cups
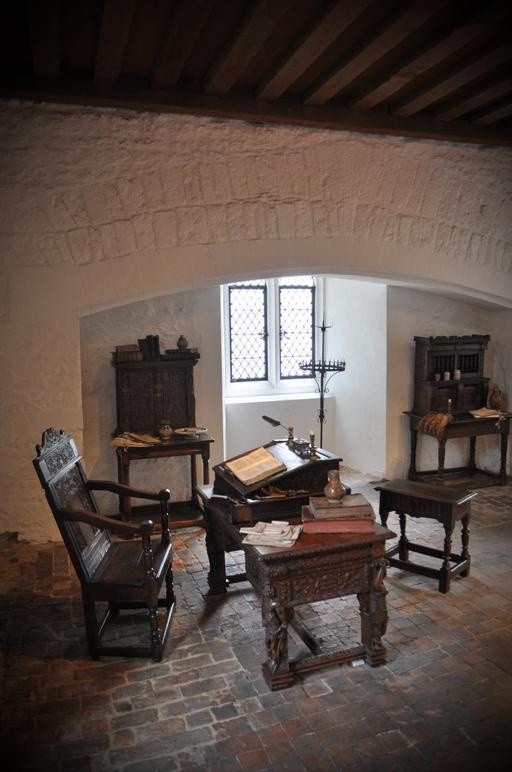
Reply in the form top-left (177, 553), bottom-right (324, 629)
top-left (434, 369), bottom-right (460, 381)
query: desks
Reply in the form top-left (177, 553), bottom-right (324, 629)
top-left (194, 484), bottom-right (398, 690)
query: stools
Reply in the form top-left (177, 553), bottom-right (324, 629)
top-left (373, 479), bottom-right (477, 596)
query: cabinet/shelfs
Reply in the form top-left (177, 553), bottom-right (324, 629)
top-left (404, 333), bottom-right (512, 490)
top-left (112, 360), bottom-right (216, 542)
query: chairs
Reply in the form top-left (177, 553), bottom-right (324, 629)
top-left (33, 425), bottom-right (178, 663)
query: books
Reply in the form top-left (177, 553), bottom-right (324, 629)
top-left (225, 446), bottom-right (287, 486)
top-left (238, 492), bottom-right (375, 548)
